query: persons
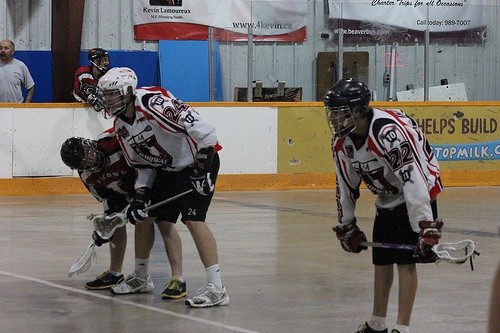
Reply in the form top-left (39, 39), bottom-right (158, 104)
top-left (323, 77), bottom-right (445, 333)
top-left (59, 126), bottom-right (189, 300)
top-left (68, 48), bottom-right (110, 104)
top-left (0, 39), bottom-right (37, 104)
top-left (96, 67), bottom-right (230, 308)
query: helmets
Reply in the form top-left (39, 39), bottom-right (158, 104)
top-left (87, 48), bottom-right (109, 73)
top-left (61, 137), bottom-right (108, 172)
top-left (95, 66), bottom-right (138, 119)
top-left (323, 78), bottom-right (371, 139)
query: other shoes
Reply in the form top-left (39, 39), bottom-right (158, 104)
top-left (351, 321), bottom-right (388, 333)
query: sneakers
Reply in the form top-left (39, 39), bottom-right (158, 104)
top-left (186, 283), bottom-right (231, 307)
top-left (109, 273), bottom-right (155, 294)
top-left (82, 270), bottom-right (125, 290)
top-left (160, 278), bottom-right (188, 299)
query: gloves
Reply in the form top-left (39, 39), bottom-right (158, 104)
top-left (415, 220), bottom-right (446, 261)
top-left (189, 147), bottom-right (217, 196)
top-left (333, 217), bottom-right (369, 254)
top-left (126, 187), bottom-right (152, 225)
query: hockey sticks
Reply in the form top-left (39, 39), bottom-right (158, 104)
top-left (68, 235), bottom-right (103, 275)
top-left (359, 240), bottom-right (476, 266)
top-left (91, 186), bottom-right (201, 239)
